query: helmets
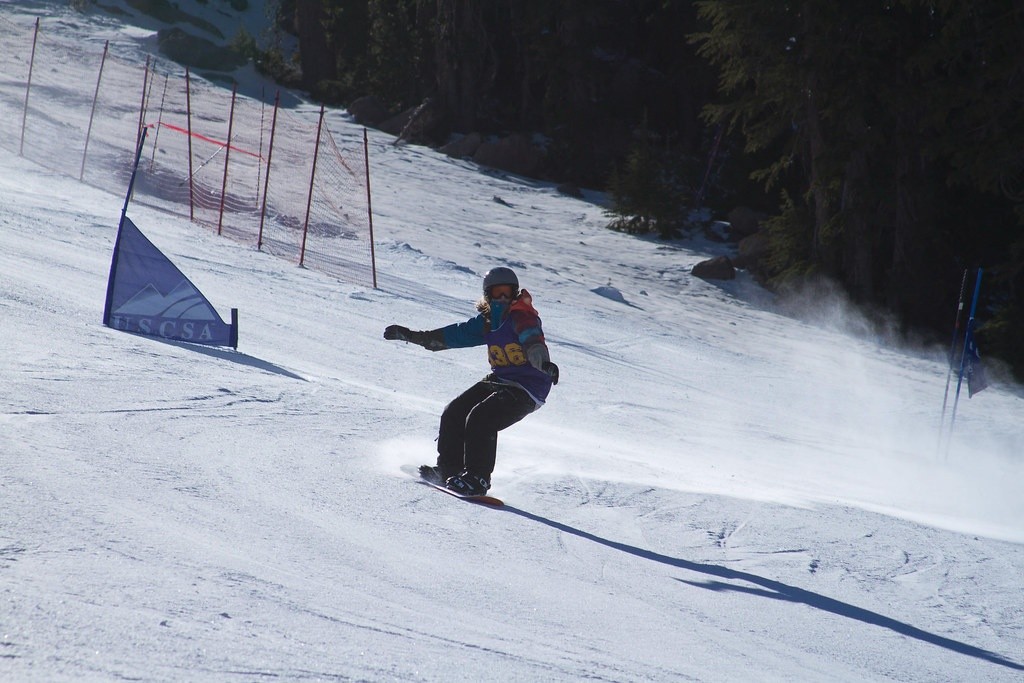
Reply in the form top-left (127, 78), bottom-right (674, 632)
top-left (483, 267), bottom-right (519, 300)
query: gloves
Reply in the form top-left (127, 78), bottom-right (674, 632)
top-left (542, 361), bottom-right (559, 385)
top-left (383, 324), bottom-right (410, 341)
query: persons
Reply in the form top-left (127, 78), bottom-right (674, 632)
top-left (383, 267), bottom-right (560, 493)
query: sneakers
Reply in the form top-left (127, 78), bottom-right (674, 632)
top-left (418, 464), bottom-right (467, 488)
top-left (445, 464), bottom-right (491, 496)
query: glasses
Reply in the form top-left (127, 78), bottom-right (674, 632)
top-left (491, 285), bottom-right (513, 299)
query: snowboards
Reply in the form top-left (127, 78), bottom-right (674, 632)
top-left (418, 465), bottom-right (506, 508)
